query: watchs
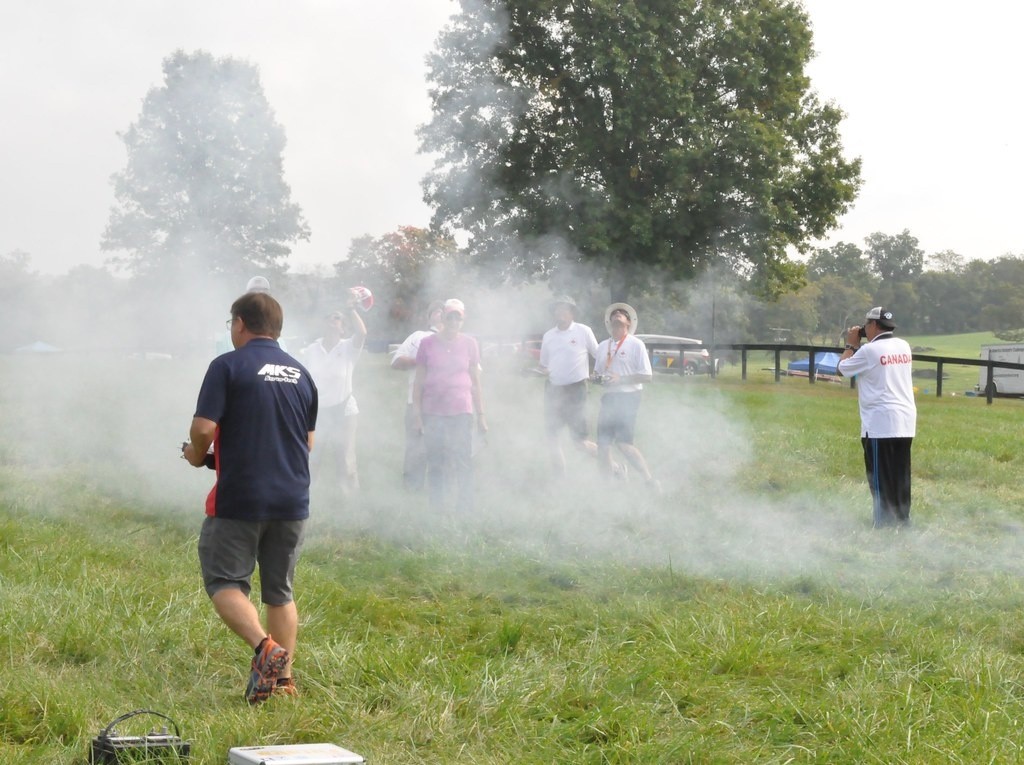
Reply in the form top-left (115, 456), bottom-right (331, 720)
top-left (844, 344), bottom-right (857, 353)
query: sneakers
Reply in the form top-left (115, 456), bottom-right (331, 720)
top-left (245, 634), bottom-right (298, 704)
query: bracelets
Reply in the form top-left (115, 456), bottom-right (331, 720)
top-left (477, 413), bottom-right (486, 416)
top-left (348, 306), bottom-right (355, 311)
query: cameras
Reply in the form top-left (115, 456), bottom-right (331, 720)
top-left (593, 374), bottom-right (609, 385)
top-left (858, 325), bottom-right (867, 338)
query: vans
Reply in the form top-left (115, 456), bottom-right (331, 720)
top-left (633, 334), bottom-right (711, 376)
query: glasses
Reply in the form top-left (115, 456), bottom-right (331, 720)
top-left (226, 318), bottom-right (239, 330)
top-left (611, 309), bottom-right (630, 320)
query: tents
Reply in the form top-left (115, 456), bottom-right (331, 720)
top-left (15, 341), bottom-right (64, 360)
top-left (786, 351), bottom-right (844, 385)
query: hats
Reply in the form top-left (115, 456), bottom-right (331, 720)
top-left (351, 286), bottom-right (374, 312)
top-left (443, 299), bottom-right (465, 318)
top-left (248, 276), bottom-right (270, 292)
top-left (551, 295), bottom-right (582, 321)
top-left (866, 306), bottom-right (899, 328)
top-left (604, 303), bottom-right (638, 337)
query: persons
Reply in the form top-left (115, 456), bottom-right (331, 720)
top-left (839, 306), bottom-right (918, 532)
top-left (538, 294), bottom-right (629, 484)
top-left (388, 300), bottom-right (484, 493)
top-left (246, 276), bottom-right (287, 352)
top-left (409, 299), bottom-right (488, 511)
top-left (294, 294), bottom-right (368, 483)
top-left (179, 293), bottom-right (319, 704)
top-left (590, 302), bottom-right (661, 494)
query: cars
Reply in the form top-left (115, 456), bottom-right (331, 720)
top-left (513, 338), bottom-right (544, 359)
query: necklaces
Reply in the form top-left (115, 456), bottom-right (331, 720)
top-left (447, 349), bottom-right (450, 352)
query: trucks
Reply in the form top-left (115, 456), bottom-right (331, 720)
top-left (977, 343), bottom-right (1024, 397)
